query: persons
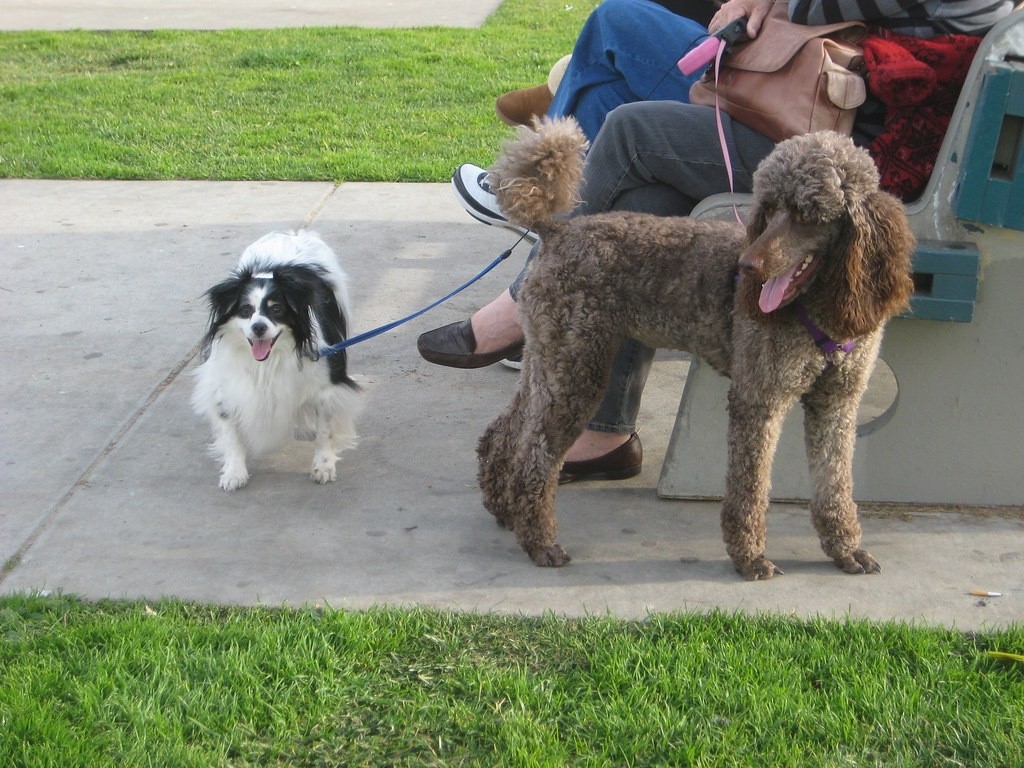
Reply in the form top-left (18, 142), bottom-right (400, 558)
top-left (415, 0), bottom-right (1024, 487)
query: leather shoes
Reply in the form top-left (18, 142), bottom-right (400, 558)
top-left (558, 432), bottom-right (642, 485)
top-left (417, 317), bottom-right (524, 369)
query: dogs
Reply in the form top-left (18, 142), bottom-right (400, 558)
top-left (189, 232), bottom-right (362, 492)
top-left (474, 115), bottom-right (917, 583)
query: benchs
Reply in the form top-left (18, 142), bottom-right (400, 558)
top-left (656, 0), bottom-right (1024, 509)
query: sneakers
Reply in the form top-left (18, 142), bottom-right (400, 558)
top-left (452, 164), bottom-right (539, 246)
top-left (495, 83), bottom-right (554, 130)
top-left (501, 348), bottom-right (523, 368)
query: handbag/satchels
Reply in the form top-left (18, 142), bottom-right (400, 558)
top-left (689, 1), bottom-right (867, 142)
